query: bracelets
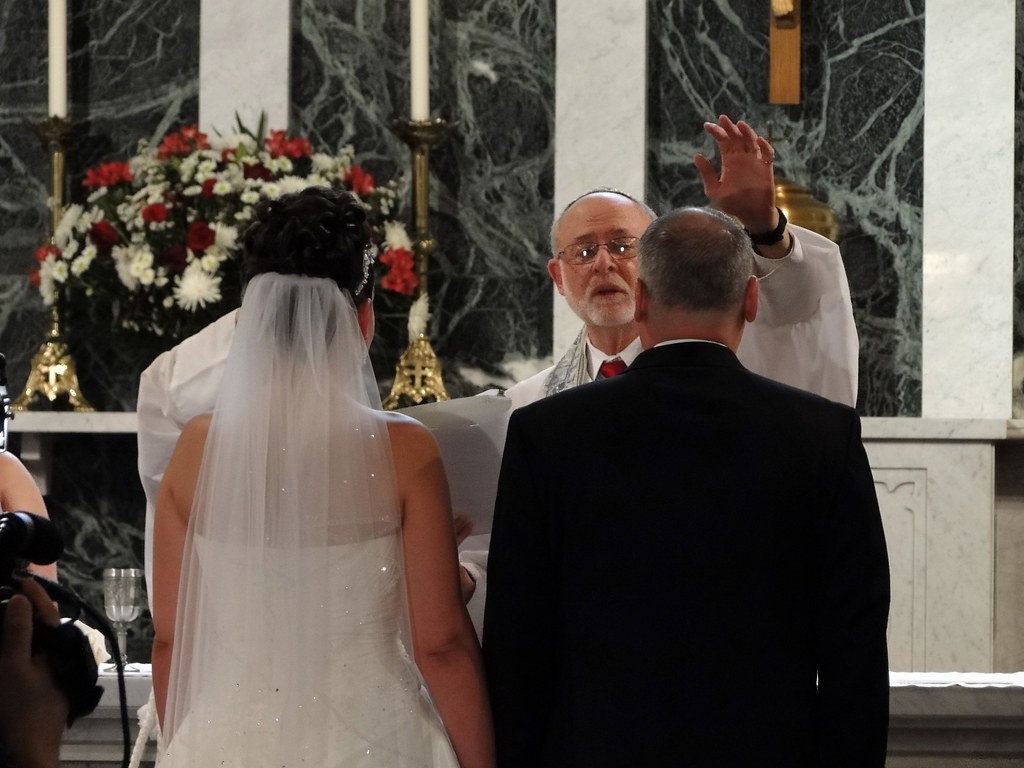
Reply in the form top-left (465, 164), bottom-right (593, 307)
top-left (743, 204), bottom-right (787, 247)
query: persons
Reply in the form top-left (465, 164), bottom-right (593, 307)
top-left (482, 203), bottom-right (892, 768)
top-left (149, 184), bottom-right (494, 768)
top-left (455, 114), bottom-right (862, 656)
top-left (0, 437), bottom-right (72, 768)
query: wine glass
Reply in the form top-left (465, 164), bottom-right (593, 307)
top-left (102, 568), bottom-right (142, 672)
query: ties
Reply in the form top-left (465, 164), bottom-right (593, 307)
top-left (599, 359), bottom-right (628, 379)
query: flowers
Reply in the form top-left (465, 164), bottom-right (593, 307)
top-left (28, 109), bottom-right (421, 345)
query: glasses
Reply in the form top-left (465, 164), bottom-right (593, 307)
top-left (556, 234), bottom-right (642, 264)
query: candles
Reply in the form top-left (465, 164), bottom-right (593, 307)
top-left (47, 0), bottom-right (69, 119)
top-left (412, 0), bottom-right (431, 122)
